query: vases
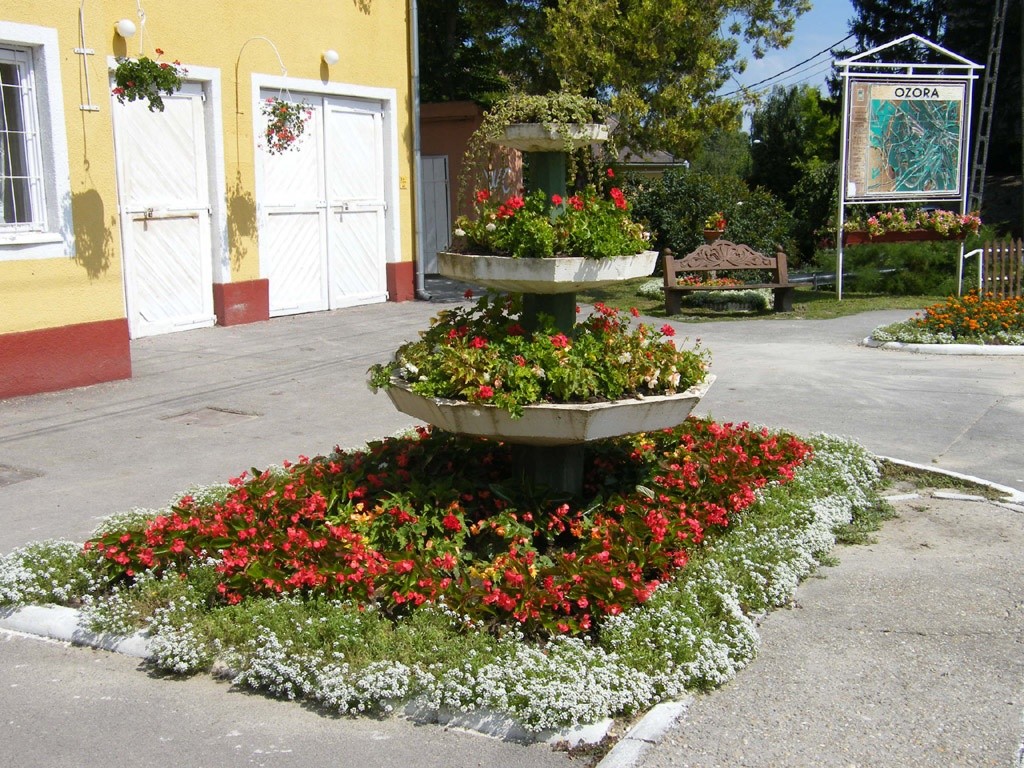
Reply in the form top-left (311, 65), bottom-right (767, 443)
top-left (438, 248), bottom-right (659, 294)
top-left (703, 230), bottom-right (724, 244)
top-left (831, 227), bottom-right (967, 245)
top-left (384, 372), bottom-right (715, 445)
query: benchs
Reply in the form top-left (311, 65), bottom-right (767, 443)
top-left (664, 241), bottom-right (813, 317)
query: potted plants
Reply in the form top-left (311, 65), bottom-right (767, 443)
top-left (458, 92), bottom-right (621, 187)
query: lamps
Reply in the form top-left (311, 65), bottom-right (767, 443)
top-left (114, 19), bottom-right (135, 38)
top-left (321, 50), bottom-right (338, 65)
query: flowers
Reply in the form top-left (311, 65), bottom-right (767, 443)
top-left (366, 289), bottom-right (714, 418)
top-left (111, 48), bottom-right (188, 114)
top-left (705, 211), bottom-right (726, 230)
top-left (450, 168), bottom-right (651, 259)
top-left (815, 209), bottom-right (981, 249)
top-left (262, 95), bottom-right (316, 153)
